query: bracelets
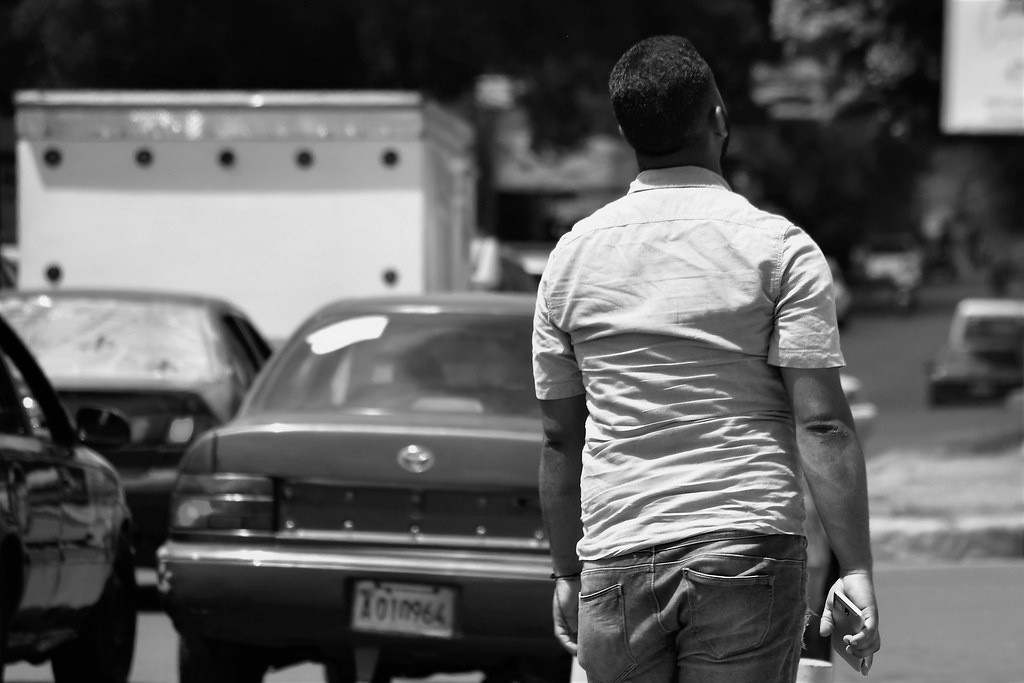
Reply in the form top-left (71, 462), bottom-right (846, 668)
top-left (550, 573), bottom-right (581, 580)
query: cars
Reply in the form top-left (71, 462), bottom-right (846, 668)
top-left (0, 312), bottom-right (140, 683)
top-left (153, 288), bottom-right (575, 683)
top-left (0, 285), bottom-right (279, 539)
top-left (917, 295), bottom-right (1022, 404)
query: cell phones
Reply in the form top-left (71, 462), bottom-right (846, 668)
top-left (829, 591), bottom-right (865, 672)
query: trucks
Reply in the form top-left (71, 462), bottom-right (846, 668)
top-left (0, 87), bottom-right (505, 354)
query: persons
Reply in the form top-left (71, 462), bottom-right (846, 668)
top-left (533, 36), bottom-right (882, 683)
